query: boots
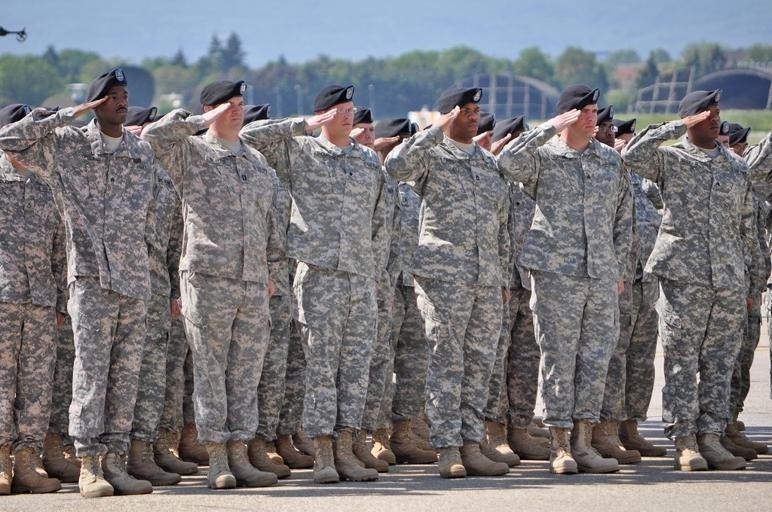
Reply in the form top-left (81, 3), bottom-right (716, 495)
top-left (673, 412), bottom-right (768, 472)
top-left (312, 410), bottom-right (439, 482)
top-left (435, 412), bottom-right (550, 477)
top-left (0, 432), bottom-right (82, 494)
top-left (204, 427), bottom-right (315, 487)
top-left (78, 422), bottom-right (209, 498)
top-left (549, 416), bottom-right (667, 475)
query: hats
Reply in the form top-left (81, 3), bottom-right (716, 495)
top-left (241, 103), bottom-right (273, 124)
top-left (199, 79), bottom-right (247, 107)
top-left (87, 68), bottom-right (128, 103)
top-left (436, 86), bottom-right (483, 116)
top-left (0, 103), bottom-right (60, 130)
top-left (678, 88), bottom-right (721, 120)
top-left (476, 111), bottom-right (530, 141)
top-left (313, 83), bottom-right (354, 113)
top-left (126, 103), bottom-right (158, 127)
top-left (555, 84), bottom-right (600, 117)
top-left (352, 107), bottom-right (419, 140)
top-left (717, 119), bottom-right (752, 145)
top-left (597, 104), bottom-right (638, 136)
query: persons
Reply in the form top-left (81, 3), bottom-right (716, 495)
top-left (0, 69), bottom-right (771, 499)
